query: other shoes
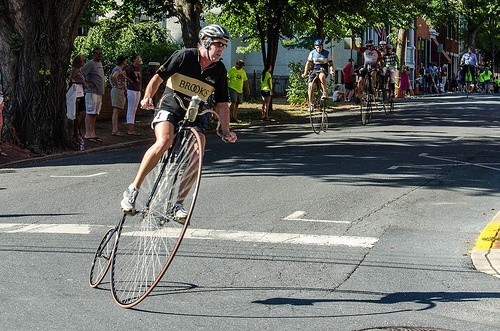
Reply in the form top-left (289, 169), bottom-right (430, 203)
top-left (308, 105), bottom-right (314, 111)
top-left (128, 130), bottom-right (141, 136)
top-left (320, 93), bottom-right (327, 101)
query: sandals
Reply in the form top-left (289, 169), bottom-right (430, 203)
top-left (112, 131), bottom-right (127, 137)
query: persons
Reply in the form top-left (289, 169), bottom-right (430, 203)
top-left (260, 63), bottom-right (272, 120)
top-left (121, 24), bottom-right (237, 219)
top-left (353, 40), bottom-right (382, 97)
top-left (460, 46), bottom-right (477, 84)
top-left (80, 47), bottom-right (105, 143)
top-left (419, 64), bottom-right (500, 95)
top-left (71, 54), bottom-right (86, 145)
top-left (111, 55), bottom-right (128, 136)
top-left (379, 40), bottom-right (386, 68)
top-left (125, 53), bottom-right (143, 136)
top-left (227, 60), bottom-right (249, 122)
top-left (301, 39), bottom-right (335, 107)
top-left (399, 65), bottom-right (409, 96)
top-left (382, 44), bottom-right (399, 97)
top-left (342, 58), bottom-right (356, 102)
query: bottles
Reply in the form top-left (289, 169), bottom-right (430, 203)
top-left (368, 61), bottom-right (371, 70)
top-left (185, 94), bottom-right (201, 121)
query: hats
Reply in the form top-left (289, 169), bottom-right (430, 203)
top-left (348, 58), bottom-right (354, 62)
top-left (238, 60), bottom-right (245, 66)
top-left (366, 39), bottom-right (375, 46)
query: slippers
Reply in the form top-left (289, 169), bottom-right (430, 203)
top-left (83, 137), bottom-right (97, 143)
top-left (93, 137), bottom-right (103, 142)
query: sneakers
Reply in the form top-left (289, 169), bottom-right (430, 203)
top-left (121, 184), bottom-right (139, 212)
top-left (170, 206), bottom-right (188, 219)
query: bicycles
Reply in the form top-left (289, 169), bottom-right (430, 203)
top-left (352, 65), bottom-right (377, 127)
top-left (88, 92), bottom-right (235, 308)
top-left (460, 65), bottom-right (480, 99)
top-left (301, 68), bottom-right (335, 135)
top-left (373, 66), bottom-right (398, 119)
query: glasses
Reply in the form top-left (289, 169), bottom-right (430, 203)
top-left (212, 42), bottom-right (228, 49)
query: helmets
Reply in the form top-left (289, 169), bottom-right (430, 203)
top-left (379, 41), bottom-right (386, 45)
top-left (314, 40), bottom-right (322, 47)
top-left (199, 25), bottom-right (230, 42)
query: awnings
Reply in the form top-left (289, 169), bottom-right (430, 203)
top-left (431, 38), bottom-right (452, 64)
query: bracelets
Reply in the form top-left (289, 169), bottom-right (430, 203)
top-left (225, 128), bottom-right (232, 136)
